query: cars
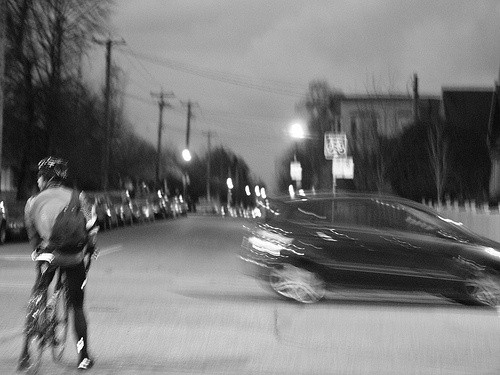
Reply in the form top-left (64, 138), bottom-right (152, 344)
top-left (239, 192), bottom-right (499, 308)
top-left (0, 191), bottom-right (189, 246)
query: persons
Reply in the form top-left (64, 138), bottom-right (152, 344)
top-left (14, 153), bottom-right (98, 373)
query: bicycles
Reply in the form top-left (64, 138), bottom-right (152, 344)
top-left (22, 224), bottom-right (100, 375)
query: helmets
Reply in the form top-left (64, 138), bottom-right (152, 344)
top-left (37, 156), bottom-right (69, 171)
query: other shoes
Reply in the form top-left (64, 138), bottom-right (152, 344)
top-left (77, 356), bottom-right (95, 369)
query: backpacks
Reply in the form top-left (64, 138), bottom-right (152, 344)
top-left (49, 187), bottom-right (87, 254)
